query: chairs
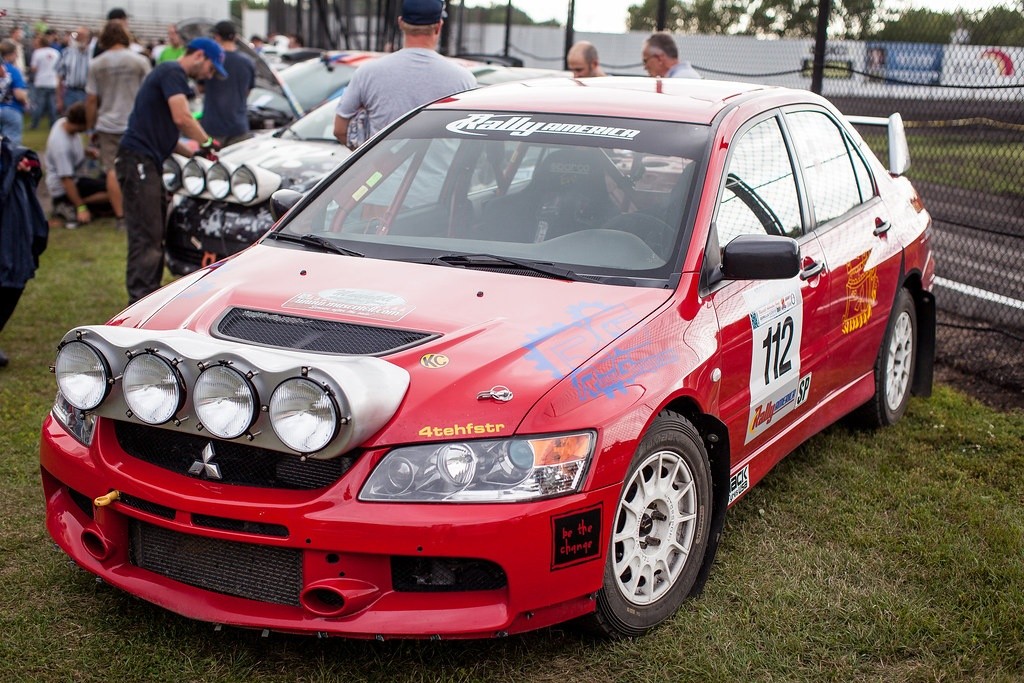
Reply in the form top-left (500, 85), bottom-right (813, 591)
top-left (667, 162), bottom-right (703, 230)
top-left (473, 150), bottom-right (616, 248)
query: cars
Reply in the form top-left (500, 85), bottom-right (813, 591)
top-left (244, 42), bottom-right (388, 127)
top-left (37, 77), bottom-right (937, 641)
top-left (160, 66), bottom-right (580, 278)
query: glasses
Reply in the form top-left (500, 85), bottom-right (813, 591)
top-left (642, 54), bottom-right (655, 64)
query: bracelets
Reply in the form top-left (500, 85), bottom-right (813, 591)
top-left (76, 205), bottom-right (88, 212)
top-left (201, 137), bottom-right (213, 149)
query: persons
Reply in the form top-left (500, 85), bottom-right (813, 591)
top-left (198, 21), bottom-right (257, 151)
top-left (0, 131), bottom-right (30, 370)
top-left (0, 9), bottom-right (205, 231)
top-left (118, 37), bottom-right (229, 306)
top-left (642, 34), bottom-right (703, 79)
top-left (334, 0), bottom-right (478, 222)
top-left (252, 31), bottom-right (304, 54)
top-left (567, 41), bottom-right (608, 78)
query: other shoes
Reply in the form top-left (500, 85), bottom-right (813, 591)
top-left (111, 219), bottom-right (125, 229)
top-left (55, 204), bottom-right (77, 221)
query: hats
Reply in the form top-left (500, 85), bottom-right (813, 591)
top-left (187, 37), bottom-right (229, 80)
top-left (402, 1), bottom-right (445, 26)
